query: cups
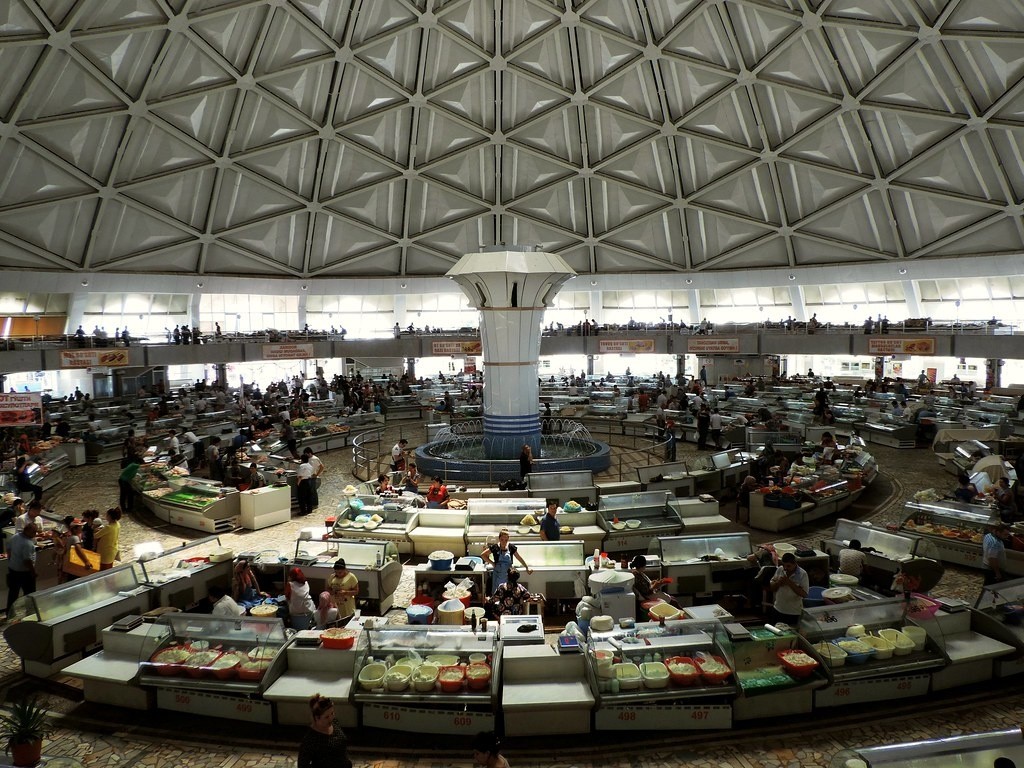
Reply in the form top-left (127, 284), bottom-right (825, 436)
top-left (354, 609), bottom-right (361, 619)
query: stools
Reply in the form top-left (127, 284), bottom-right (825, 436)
top-left (736, 500), bottom-right (750, 522)
top-left (524, 600), bottom-right (541, 615)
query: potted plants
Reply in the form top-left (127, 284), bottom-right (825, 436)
top-left (0, 697), bottom-right (58, 768)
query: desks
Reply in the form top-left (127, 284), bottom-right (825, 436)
top-left (0, 377), bottom-right (1024, 736)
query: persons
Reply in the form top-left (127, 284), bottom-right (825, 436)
top-left (988, 316), bottom-right (997, 325)
top-left (765, 313), bottom-right (818, 334)
top-left (543, 316), bottom-right (712, 336)
top-left (473, 730), bottom-right (509, 768)
top-left (748, 544), bottom-right (781, 605)
top-left (629, 556), bottom-right (655, 622)
top-left (298, 694), bottom-right (352, 768)
top-left (484, 569), bottom-right (534, 616)
top-left (770, 553), bottom-right (809, 627)
top-left (394, 321), bottom-right (436, 338)
top-left (74, 322), bottom-right (347, 347)
top-left (0, 368), bottom-right (483, 620)
top-left (540, 502), bottom-right (560, 541)
top-left (539, 365), bottom-right (1024, 521)
top-left (983, 524), bottom-right (1009, 586)
top-left (862, 314), bottom-right (889, 335)
top-left (520, 444), bottom-right (532, 482)
top-left (481, 528), bottom-right (533, 595)
top-left (231, 559), bottom-right (359, 628)
top-left (837, 539), bottom-right (868, 585)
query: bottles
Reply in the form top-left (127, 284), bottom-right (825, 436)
top-left (423, 582), bottom-right (427, 596)
top-left (658, 615), bottom-right (666, 627)
top-left (481, 618), bottom-right (488, 631)
top-left (613, 652), bottom-right (662, 666)
top-left (595, 676), bottom-right (620, 693)
top-left (380, 487), bottom-right (402, 498)
top-left (471, 609), bottom-right (477, 630)
top-left (418, 583), bottom-right (422, 596)
top-left (621, 553), bottom-right (628, 568)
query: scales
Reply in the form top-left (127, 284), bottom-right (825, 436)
top-left (293, 555), bottom-right (318, 566)
top-left (455, 557), bottom-right (476, 571)
top-left (935, 597), bottom-right (968, 613)
top-left (699, 493), bottom-right (716, 502)
top-left (726, 623), bottom-right (755, 641)
top-left (109, 614), bottom-right (145, 633)
top-left (558, 635), bottom-right (584, 654)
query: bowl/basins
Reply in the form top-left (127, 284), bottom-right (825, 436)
top-left (451, 575), bottom-right (473, 583)
top-left (338, 516), bottom-right (383, 529)
top-left (428, 553), bottom-right (454, 569)
top-left (610, 519), bottom-right (642, 529)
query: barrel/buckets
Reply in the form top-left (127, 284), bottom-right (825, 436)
top-left (592, 650), bottom-right (615, 677)
top-left (469, 653), bottom-right (492, 666)
top-left (902, 626), bottom-right (926, 651)
top-left (801, 585), bottom-right (830, 607)
top-left (250, 604), bottom-right (278, 618)
top-left (406, 590), bottom-right (486, 625)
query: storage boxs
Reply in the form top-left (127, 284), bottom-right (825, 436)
top-left (62, 546), bottom-right (101, 576)
